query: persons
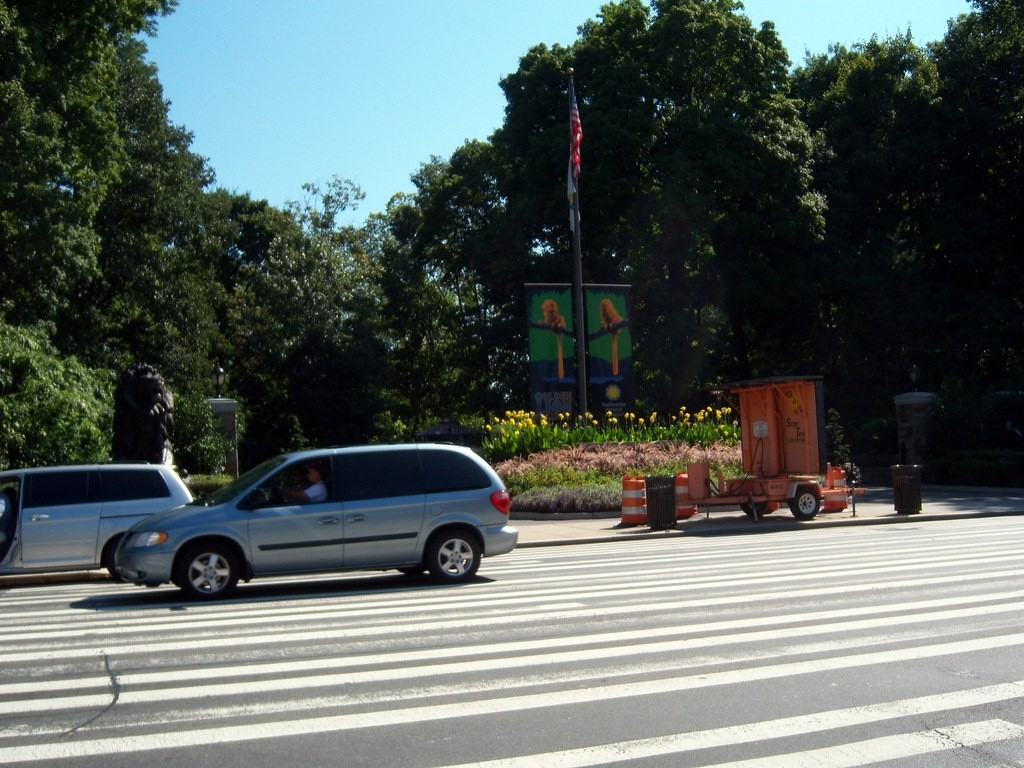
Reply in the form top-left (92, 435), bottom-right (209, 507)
top-left (0, 486), bottom-right (17, 543)
top-left (286, 461), bottom-right (327, 502)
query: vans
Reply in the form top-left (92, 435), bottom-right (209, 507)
top-left (114, 442), bottom-right (518, 598)
top-left (0, 460), bottom-right (194, 581)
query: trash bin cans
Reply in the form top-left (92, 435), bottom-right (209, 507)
top-left (891, 464), bottom-right (923, 515)
top-left (643, 474), bottom-right (678, 531)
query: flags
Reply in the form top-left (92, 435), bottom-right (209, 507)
top-left (570, 79), bottom-right (583, 179)
top-left (567, 160), bottom-right (580, 232)
